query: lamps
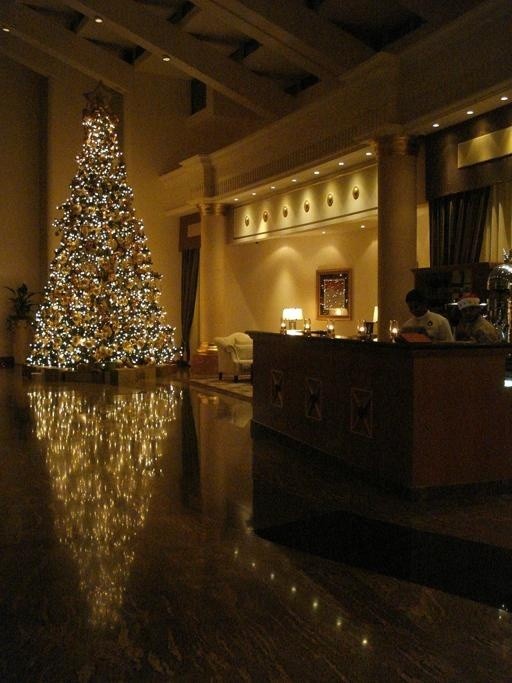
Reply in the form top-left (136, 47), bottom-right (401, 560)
top-left (214, 331), bottom-right (255, 383)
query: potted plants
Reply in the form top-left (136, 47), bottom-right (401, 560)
top-left (315, 267), bottom-right (353, 322)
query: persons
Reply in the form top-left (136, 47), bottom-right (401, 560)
top-left (398, 288), bottom-right (454, 342)
top-left (457, 290), bottom-right (497, 342)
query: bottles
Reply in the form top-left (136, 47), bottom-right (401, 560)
top-left (457, 297), bottom-right (481, 310)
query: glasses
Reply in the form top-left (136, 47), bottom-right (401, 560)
top-left (283, 306), bottom-right (304, 329)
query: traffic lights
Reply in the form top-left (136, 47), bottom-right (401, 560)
top-left (234, 336), bottom-right (254, 361)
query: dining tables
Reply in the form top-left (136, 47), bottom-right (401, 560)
top-left (3, 280), bottom-right (44, 363)
